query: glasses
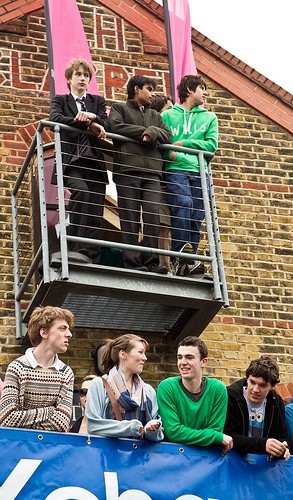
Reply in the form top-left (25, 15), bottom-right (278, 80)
top-left (79, 388), bottom-right (88, 397)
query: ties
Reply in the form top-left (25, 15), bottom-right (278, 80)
top-left (75, 96), bottom-right (91, 158)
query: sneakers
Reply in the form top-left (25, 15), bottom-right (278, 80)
top-left (133, 266), bottom-right (149, 272)
top-left (152, 265), bottom-right (168, 274)
top-left (174, 243), bottom-right (206, 274)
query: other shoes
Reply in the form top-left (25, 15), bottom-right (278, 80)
top-left (79, 248), bottom-right (90, 256)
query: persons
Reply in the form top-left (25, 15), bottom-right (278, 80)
top-left (0, 305), bottom-right (165, 442)
top-left (157, 336), bottom-right (233, 454)
top-left (49, 59), bottom-right (218, 277)
top-left (224, 357), bottom-right (293, 462)
